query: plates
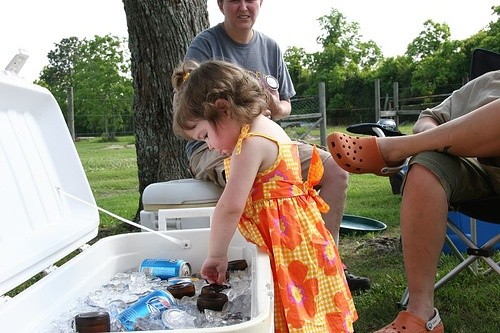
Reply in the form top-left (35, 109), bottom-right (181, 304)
top-left (340, 214), bottom-right (387, 236)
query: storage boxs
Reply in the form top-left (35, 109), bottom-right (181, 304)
top-left (0, 78), bottom-right (275, 333)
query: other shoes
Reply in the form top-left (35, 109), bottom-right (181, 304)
top-left (342, 264), bottom-right (372, 292)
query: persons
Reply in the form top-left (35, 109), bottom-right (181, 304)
top-left (327, 68), bottom-right (500, 333)
top-left (172, 59), bottom-right (359, 332)
top-left (181, 0), bottom-right (372, 291)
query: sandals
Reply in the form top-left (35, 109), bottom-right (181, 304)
top-left (367, 308), bottom-right (445, 333)
top-left (326, 132), bottom-right (408, 176)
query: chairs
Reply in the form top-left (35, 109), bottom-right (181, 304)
top-left (346, 49), bottom-right (500, 309)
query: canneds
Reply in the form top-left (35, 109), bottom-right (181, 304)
top-left (139, 258), bottom-right (192, 280)
top-left (262, 75), bottom-right (279, 91)
top-left (115, 288), bottom-right (178, 331)
top-left (167, 278), bottom-right (192, 285)
